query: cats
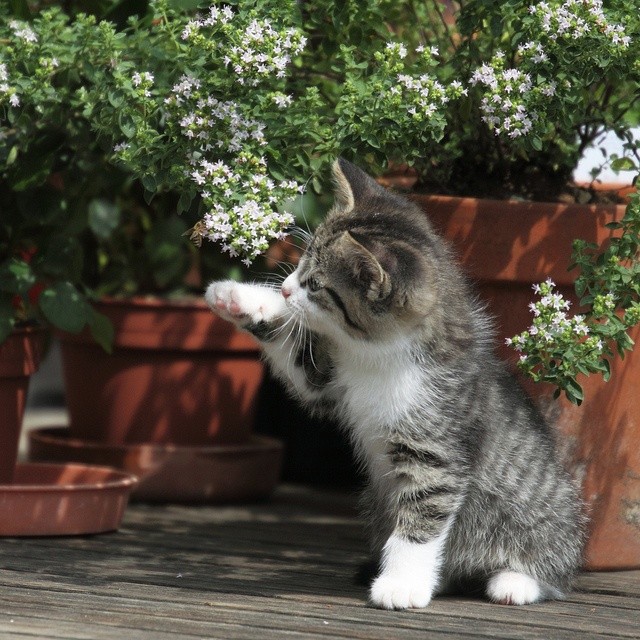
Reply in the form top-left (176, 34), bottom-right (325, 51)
top-left (202, 157), bottom-right (594, 611)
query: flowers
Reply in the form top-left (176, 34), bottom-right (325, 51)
top-left (114, 0), bottom-right (468, 296)
top-left (369, 3), bottom-right (637, 406)
top-left (0, 7), bottom-right (304, 323)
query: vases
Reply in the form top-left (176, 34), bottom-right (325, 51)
top-left (1, 321), bottom-right (52, 484)
top-left (52, 292), bottom-right (267, 446)
top-left (399, 181), bottom-right (639, 573)
